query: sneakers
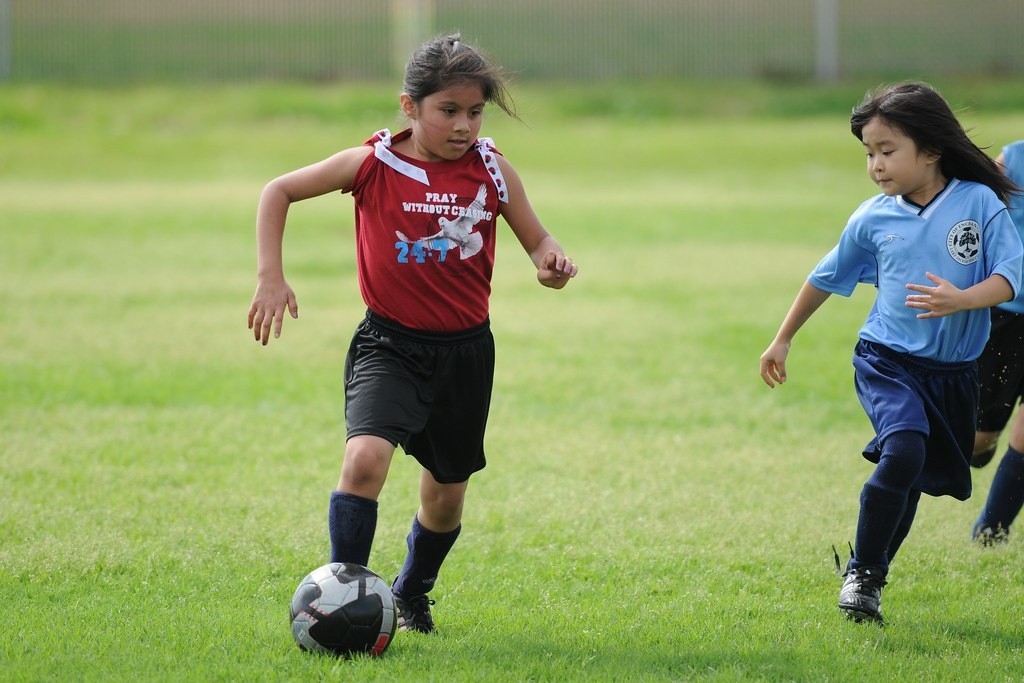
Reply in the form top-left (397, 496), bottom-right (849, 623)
top-left (390, 574), bottom-right (437, 639)
top-left (830, 542), bottom-right (884, 624)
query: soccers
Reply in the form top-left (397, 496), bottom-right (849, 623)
top-left (290, 561), bottom-right (399, 662)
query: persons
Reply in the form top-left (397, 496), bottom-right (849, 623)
top-left (248, 35), bottom-right (578, 634)
top-left (969, 142), bottom-right (1024, 545)
top-left (761, 80), bottom-right (1024, 628)
